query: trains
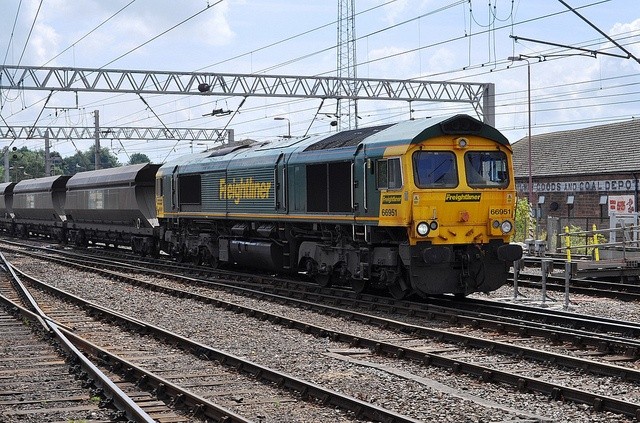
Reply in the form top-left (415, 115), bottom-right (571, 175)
top-left (0, 113), bottom-right (524, 302)
